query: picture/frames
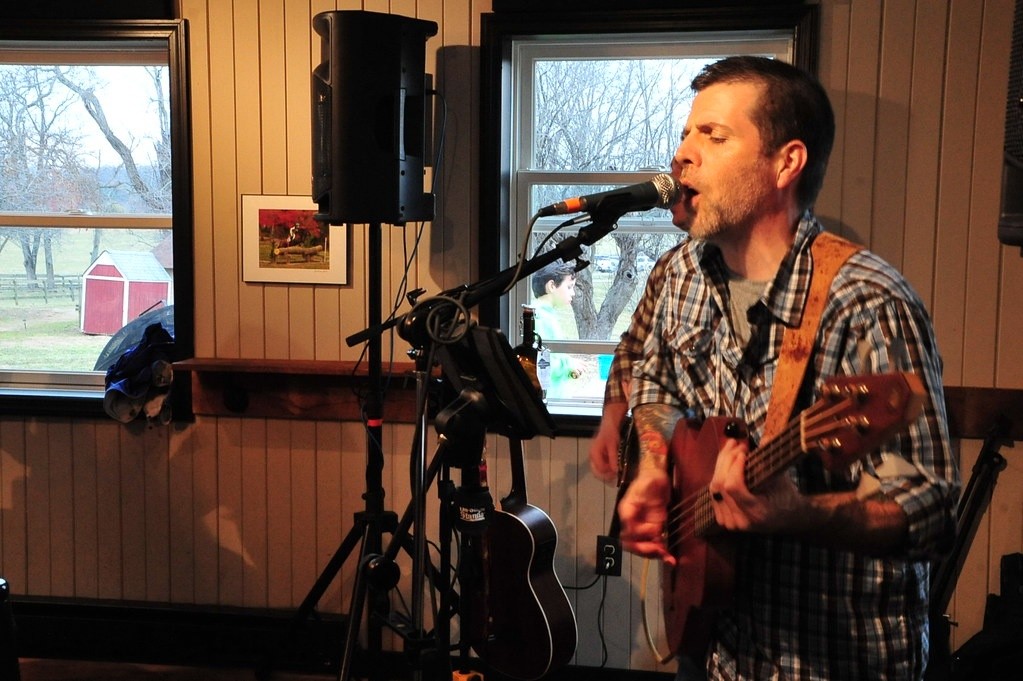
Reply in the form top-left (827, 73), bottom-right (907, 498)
top-left (241, 194), bottom-right (349, 286)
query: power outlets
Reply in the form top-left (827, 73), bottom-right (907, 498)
top-left (595, 536), bottom-right (622, 576)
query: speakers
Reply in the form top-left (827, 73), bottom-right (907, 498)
top-left (310, 10), bottom-right (438, 226)
top-left (998, 0), bottom-right (1023, 257)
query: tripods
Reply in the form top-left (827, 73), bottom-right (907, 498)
top-left (254, 221), bottom-right (468, 681)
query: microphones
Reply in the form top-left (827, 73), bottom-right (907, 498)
top-left (540, 173), bottom-right (677, 217)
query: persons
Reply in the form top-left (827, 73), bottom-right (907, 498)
top-left (532, 261), bottom-right (587, 388)
top-left (589, 53), bottom-right (962, 681)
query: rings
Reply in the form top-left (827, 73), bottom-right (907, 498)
top-left (713, 493), bottom-right (723, 502)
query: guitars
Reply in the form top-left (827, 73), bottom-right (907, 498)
top-left (657, 372), bottom-right (927, 667)
top-left (459, 303), bottom-right (580, 681)
